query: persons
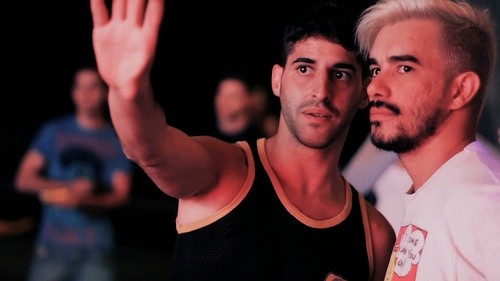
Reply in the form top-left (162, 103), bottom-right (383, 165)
top-left (16, 66), bottom-right (135, 281)
top-left (90, 0), bottom-right (500, 281)
